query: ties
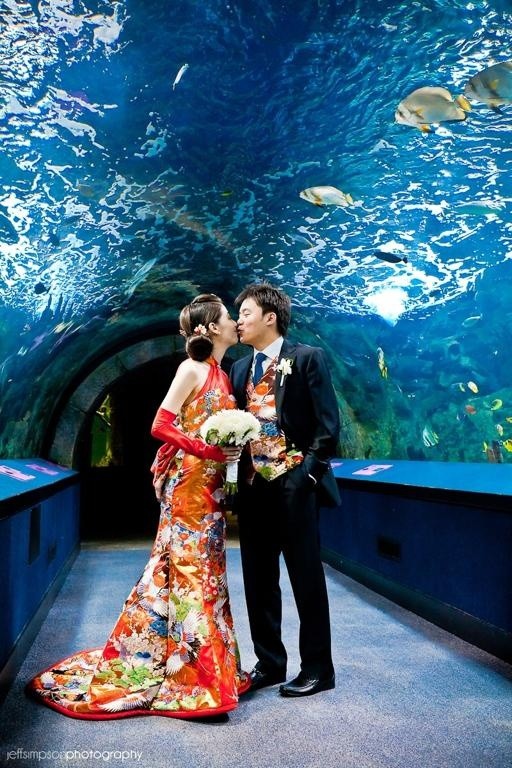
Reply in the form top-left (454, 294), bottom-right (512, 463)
top-left (252, 353), bottom-right (266, 386)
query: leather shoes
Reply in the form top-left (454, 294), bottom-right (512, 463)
top-left (249, 662), bottom-right (287, 693)
top-left (279, 670), bottom-right (335, 697)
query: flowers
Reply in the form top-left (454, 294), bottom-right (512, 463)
top-left (276, 357), bottom-right (294, 375)
top-left (198, 407), bottom-right (264, 496)
